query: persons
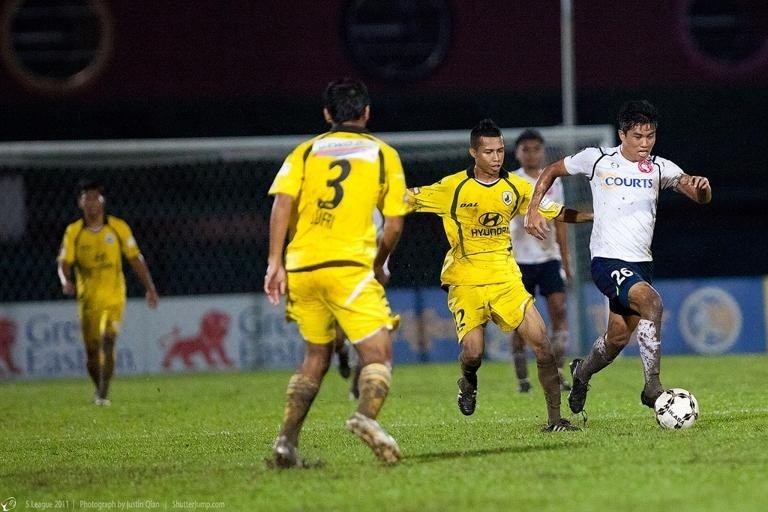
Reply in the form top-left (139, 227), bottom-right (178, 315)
top-left (335, 204), bottom-right (391, 400)
top-left (407, 120), bottom-right (594, 432)
top-left (509, 128), bottom-right (575, 393)
top-left (57, 183), bottom-right (158, 406)
top-left (523, 101), bottom-right (712, 414)
top-left (264, 75), bottom-right (408, 467)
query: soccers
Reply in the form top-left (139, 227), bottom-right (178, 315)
top-left (652, 387), bottom-right (701, 433)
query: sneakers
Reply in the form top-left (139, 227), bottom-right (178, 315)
top-left (347, 367), bottom-right (361, 400)
top-left (456, 372), bottom-right (478, 416)
top-left (269, 438), bottom-right (305, 465)
top-left (92, 391), bottom-right (113, 408)
top-left (566, 358), bottom-right (591, 414)
top-left (559, 372), bottom-right (572, 391)
top-left (345, 412), bottom-right (403, 465)
top-left (516, 378), bottom-right (534, 395)
top-left (544, 419), bottom-right (579, 435)
top-left (640, 388), bottom-right (662, 409)
top-left (337, 350), bottom-right (352, 380)
